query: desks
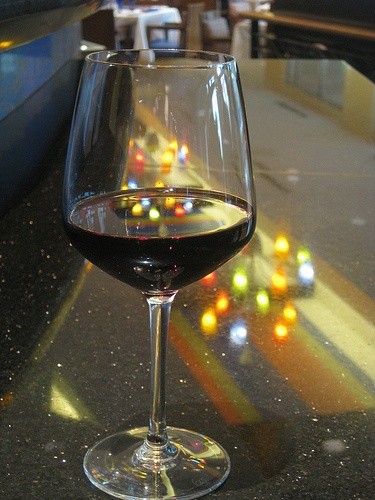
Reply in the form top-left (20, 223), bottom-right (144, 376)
top-left (1, 5), bottom-right (374, 498)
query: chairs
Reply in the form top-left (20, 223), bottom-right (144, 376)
top-left (147, 20), bottom-right (186, 56)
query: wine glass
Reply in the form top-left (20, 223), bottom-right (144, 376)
top-left (63, 49), bottom-right (257, 500)
